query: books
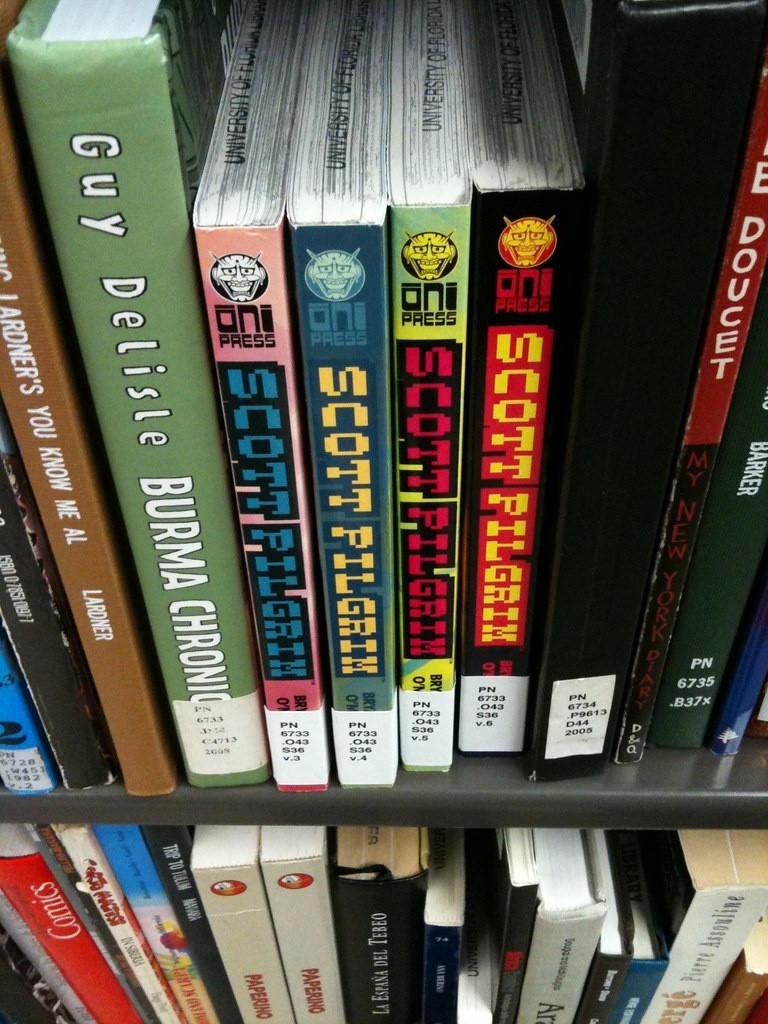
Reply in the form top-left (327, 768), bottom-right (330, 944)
top-left (0, 0), bottom-right (768, 1024)
